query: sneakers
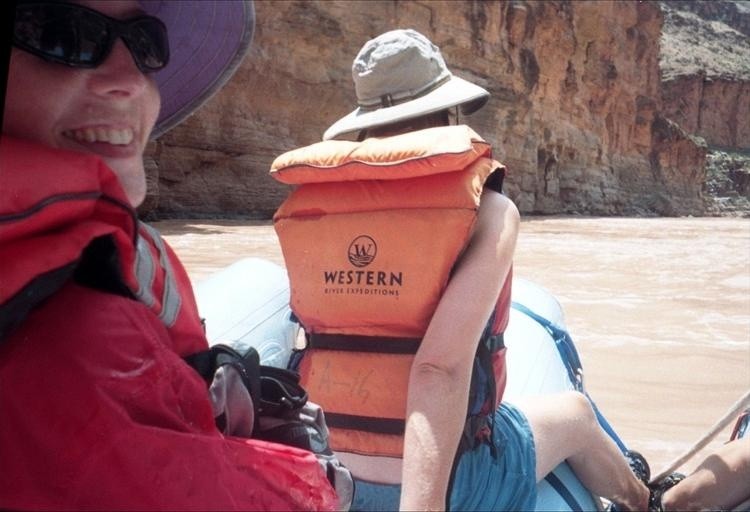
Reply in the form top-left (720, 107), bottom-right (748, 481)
top-left (606, 450), bottom-right (686, 512)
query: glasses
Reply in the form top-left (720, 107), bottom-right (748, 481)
top-left (9, 0), bottom-right (169, 72)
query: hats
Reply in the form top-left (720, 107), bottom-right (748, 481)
top-left (148, 0), bottom-right (256, 138)
top-left (321, 30), bottom-right (490, 140)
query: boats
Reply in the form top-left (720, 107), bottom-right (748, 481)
top-left (195, 258), bottom-right (604, 512)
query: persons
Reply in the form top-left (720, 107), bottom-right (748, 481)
top-left (1, 0), bottom-right (357, 512)
top-left (654, 430), bottom-right (750, 512)
top-left (268, 28), bottom-right (686, 512)
